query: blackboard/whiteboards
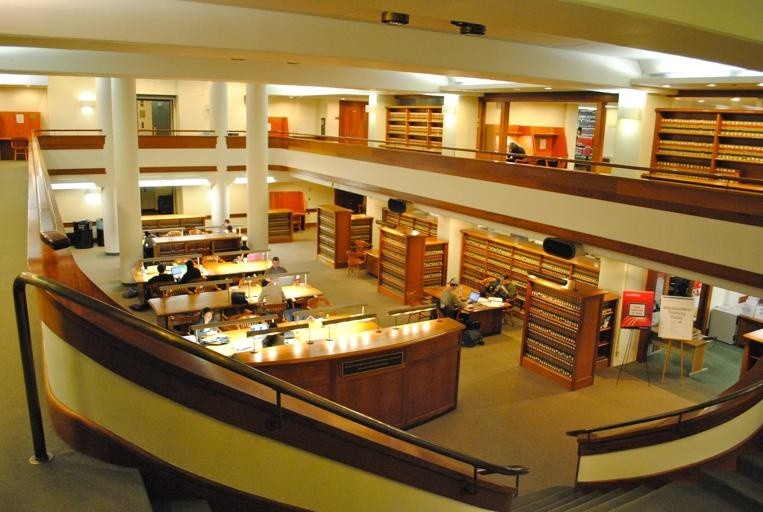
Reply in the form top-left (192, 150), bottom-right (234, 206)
top-left (658, 296), bottom-right (694, 341)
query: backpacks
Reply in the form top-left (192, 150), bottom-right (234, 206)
top-left (464, 320), bottom-right (484, 347)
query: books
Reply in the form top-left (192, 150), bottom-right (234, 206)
top-left (267, 211), bottom-right (291, 240)
top-left (349, 219), bottom-right (372, 251)
top-left (382, 209), bottom-right (436, 238)
top-left (423, 246), bottom-right (445, 287)
top-left (380, 229), bottom-right (408, 294)
top-left (461, 232), bottom-right (604, 309)
top-left (522, 281), bottom-right (582, 376)
top-left (317, 207), bottom-right (338, 262)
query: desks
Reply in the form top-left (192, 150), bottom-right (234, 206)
top-left (424, 283), bottom-right (512, 338)
top-left (181, 312), bottom-right (383, 359)
top-left (640, 321), bottom-right (709, 377)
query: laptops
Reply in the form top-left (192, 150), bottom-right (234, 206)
top-left (171, 266), bottom-right (184, 279)
top-left (274, 276), bottom-right (294, 286)
top-left (468, 290), bottom-right (481, 303)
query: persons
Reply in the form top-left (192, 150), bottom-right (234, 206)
top-left (264, 254), bottom-right (288, 275)
top-left (222, 219), bottom-right (232, 227)
top-left (506, 140), bottom-right (527, 164)
top-left (142, 229), bottom-right (155, 268)
top-left (143, 263), bottom-right (174, 299)
top-left (225, 225), bottom-right (234, 232)
top-left (257, 278), bottom-right (286, 315)
top-left (178, 259), bottom-right (202, 292)
top-left (189, 306), bottom-right (221, 337)
top-left (439, 280), bottom-right (468, 320)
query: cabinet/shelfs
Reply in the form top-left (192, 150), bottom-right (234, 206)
top-left (232, 318), bottom-right (467, 431)
top-left (458, 228), bottom-right (622, 391)
top-left (650, 108), bottom-right (763, 192)
top-left (316, 203), bottom-right (449, 306)
top-left (385, 105), bottom-right (443, 153)
top-left (141, 215), bottom-right (208, 236)
top-left (267, 209), bottom-right (293, 243)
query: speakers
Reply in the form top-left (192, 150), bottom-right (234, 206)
top-left (388, 198), bottom-right (406, 213)
top-left (543, 237), bottom-right (583, 260)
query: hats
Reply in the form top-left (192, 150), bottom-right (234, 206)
top-left (447, 277), bottom-right (458, 284)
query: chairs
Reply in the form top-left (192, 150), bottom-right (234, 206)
top-left (515, 157), bottom-right (561, 167)
top-left (501, 293), bottom-right (516, 328)
top-left (131, 228), bottom-right (336, 328)
